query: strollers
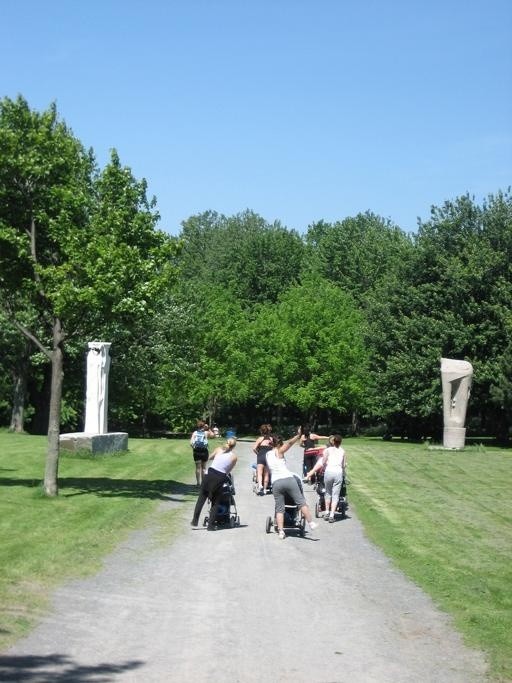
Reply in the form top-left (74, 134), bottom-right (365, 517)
top-left (202, 447), bottom-right (349, 536)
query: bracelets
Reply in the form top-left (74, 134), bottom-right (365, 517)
top-left (295, 434), bottom-right (300, 440)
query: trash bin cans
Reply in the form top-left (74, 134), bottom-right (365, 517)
top-left (226, 427), bottom-right (237, 437)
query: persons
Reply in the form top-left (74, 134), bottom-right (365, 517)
top-left (301, 433), bottom-right (335, 483)
top-left (253, 423), bottom-right (274, 495)
top-left (191, 436), bottom-right (238, 531)
top-left (264, 424), bottom-right (319, 540)
top-left (299, 423), bottom-right (333, 482)
top-left (322, 434), bottom-right (346, 522)
top-left (191, 420), bottom-right (215, 487)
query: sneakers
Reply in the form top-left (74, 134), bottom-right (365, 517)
top-left (323, 513), bottom-right (337, 523)
top-left (278, 529), bottom-right (286, 539)
top-left (190, 521), bottom-right (216, 531)
top-left (308, 521), bottom-right (319, 530)
top-left (257, 481), bottom-right (267, 496)
